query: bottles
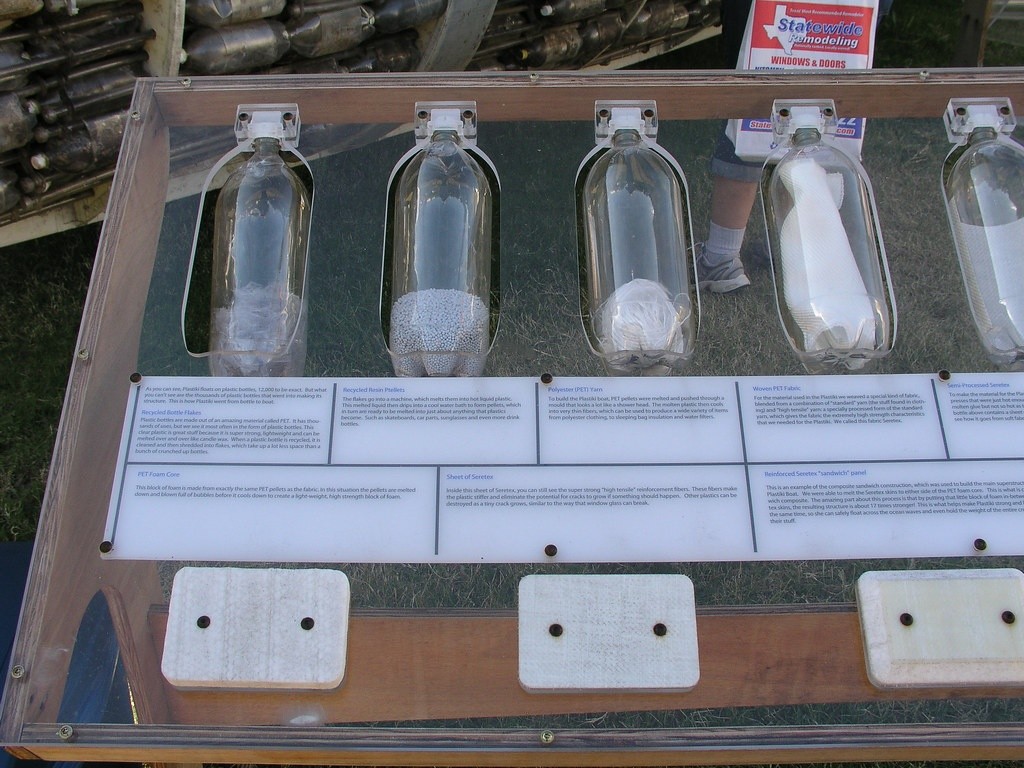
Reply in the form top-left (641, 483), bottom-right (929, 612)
top-left (944, 126), bottom-right (1024, 371)
top-left (390, 128), bottom-right (492, 376)
top-left (0, 1), bottom-right (721, 216)
top-left (768, 128), bottom-right (890, 374)
top-left (208, 137), bottom-right (309, 377)
top-left (581, 128), bottom-right (695, 377)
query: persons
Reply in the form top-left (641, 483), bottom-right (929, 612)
top-left (683, 0), bottom-right (891, 296)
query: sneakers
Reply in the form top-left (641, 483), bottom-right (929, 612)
top-left (686, 254), bottom-right (750, 295)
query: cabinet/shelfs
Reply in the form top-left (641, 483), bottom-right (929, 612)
top-left (0, 65), bottom-right (1023, 768)
